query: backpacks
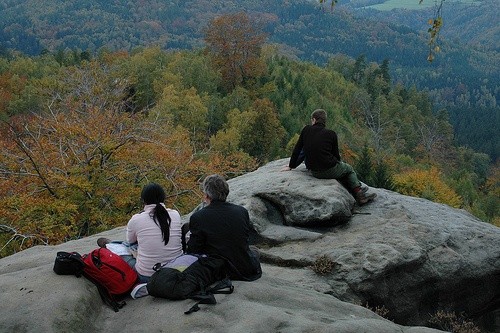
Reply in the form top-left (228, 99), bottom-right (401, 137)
top-left (80, 247), bottom-right (139, 302)
top-left (146, 253), bottom-right (226, 301)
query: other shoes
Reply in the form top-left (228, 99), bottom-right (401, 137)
top-left (361, 187), bottom-right (369, 193)
top-left (97, 237), bottom-right (112, 248)
top-left (358, 192), bottom-right (377, 206)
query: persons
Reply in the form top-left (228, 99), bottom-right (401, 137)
top-left (279, 108), bottom-right (377, 207)
top-left (97, 182), bottom-right (185, 282)
top-left (180, 175), bottom-right (263, 282)
